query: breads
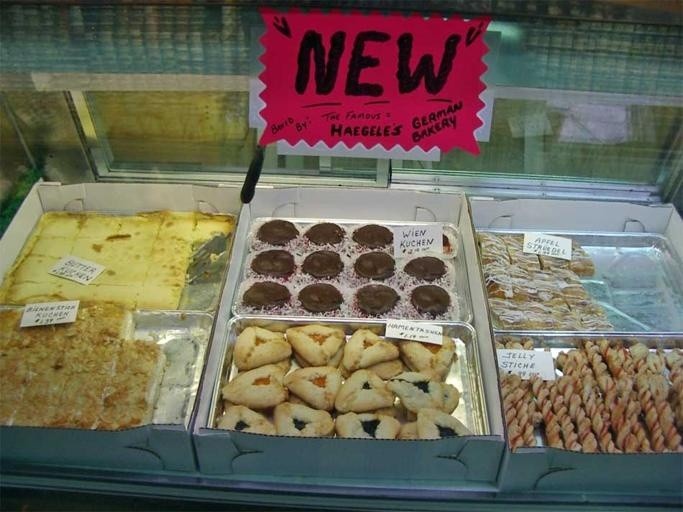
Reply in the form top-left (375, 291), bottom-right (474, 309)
top-left (477, 232), bottom-right (615, 333)
top-left (494, 337), bottom-right (683, 454)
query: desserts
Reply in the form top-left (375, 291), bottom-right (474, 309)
top-left (1, 208), bottom-right (235, 311)
top-left (1, 304), bottom-right (167, 429)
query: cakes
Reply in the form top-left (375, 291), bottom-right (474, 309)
top-left (244, 220), bottom-right (455, 317)
top-left (217, 324), bottom-right (474, 440)
